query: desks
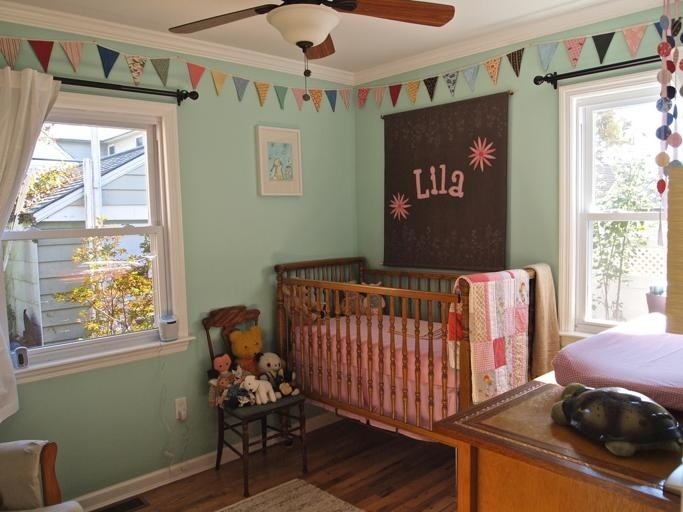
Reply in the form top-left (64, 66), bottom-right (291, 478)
top-left (434, 371), bottom-right (682, 512)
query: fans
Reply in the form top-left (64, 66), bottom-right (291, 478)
top-left (168, 0), bottom-right (455, 60)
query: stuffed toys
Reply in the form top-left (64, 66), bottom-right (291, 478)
top-left (340, 280), bottom-right (366, 314)
top-left (282, 274), bottom-right (327, 326)
top-left (361, 281), bottom-right (386, 315)
top-left (208, 325), bottom-right (301, 406)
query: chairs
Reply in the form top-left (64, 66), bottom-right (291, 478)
top-left (0, 440), bottom-right (83, 512)
top-left (202, 306), bottom-right (306, 497)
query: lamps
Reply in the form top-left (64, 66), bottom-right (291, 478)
top-left (266, 4), bottom-right (341, 101)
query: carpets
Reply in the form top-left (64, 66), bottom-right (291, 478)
top-left (214, 479), bottom-right (366, 512)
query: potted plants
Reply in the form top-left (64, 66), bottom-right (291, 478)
top-left (646, 284), bottom-right (666, 314)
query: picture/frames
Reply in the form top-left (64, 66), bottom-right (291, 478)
top-left (256, 126), bottom-right (303, 197)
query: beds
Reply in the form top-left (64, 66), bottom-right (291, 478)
top-left (273, 257), bottom-right (535, 447)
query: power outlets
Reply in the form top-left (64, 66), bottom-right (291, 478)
top-left (175, 398), bottom-right (187, 419)
